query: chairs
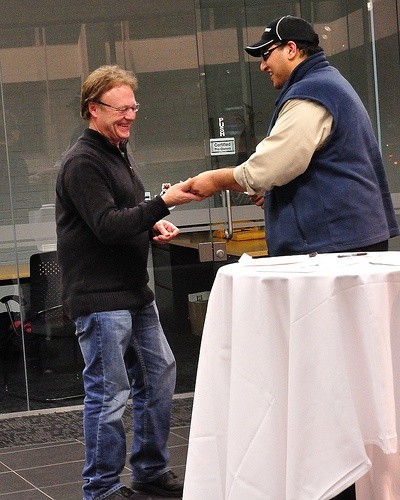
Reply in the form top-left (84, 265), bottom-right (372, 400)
top-left (0, 251), bottom-right (86, 403)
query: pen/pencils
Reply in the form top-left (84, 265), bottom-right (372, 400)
top-left (337, 253), bottom-right (367, 258)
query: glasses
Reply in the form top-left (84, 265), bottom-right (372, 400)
top-left (261, 43), bottom-right (285, 60)
top-left (91, 101), bottom-right (141, 115)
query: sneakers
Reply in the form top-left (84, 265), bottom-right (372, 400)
top-left (102, 486), bottom-right (153, 500)
top-left (132, 470), bottom-right (184, 497)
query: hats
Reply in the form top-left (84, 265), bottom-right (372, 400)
top-left (244, 14), bottom-right (320, 57)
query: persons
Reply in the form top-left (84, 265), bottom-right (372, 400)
top-left (188, 16), bottom-right (400, 258)
top-left (55, 64), bottom-right (202, 499)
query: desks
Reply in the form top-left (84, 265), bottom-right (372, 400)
top-left (152, 224), bottom-right (269, 388)
top-left (0, 262), bottom-right (31, 287)
top-left (183, 251), bottom-right (400, 500)
